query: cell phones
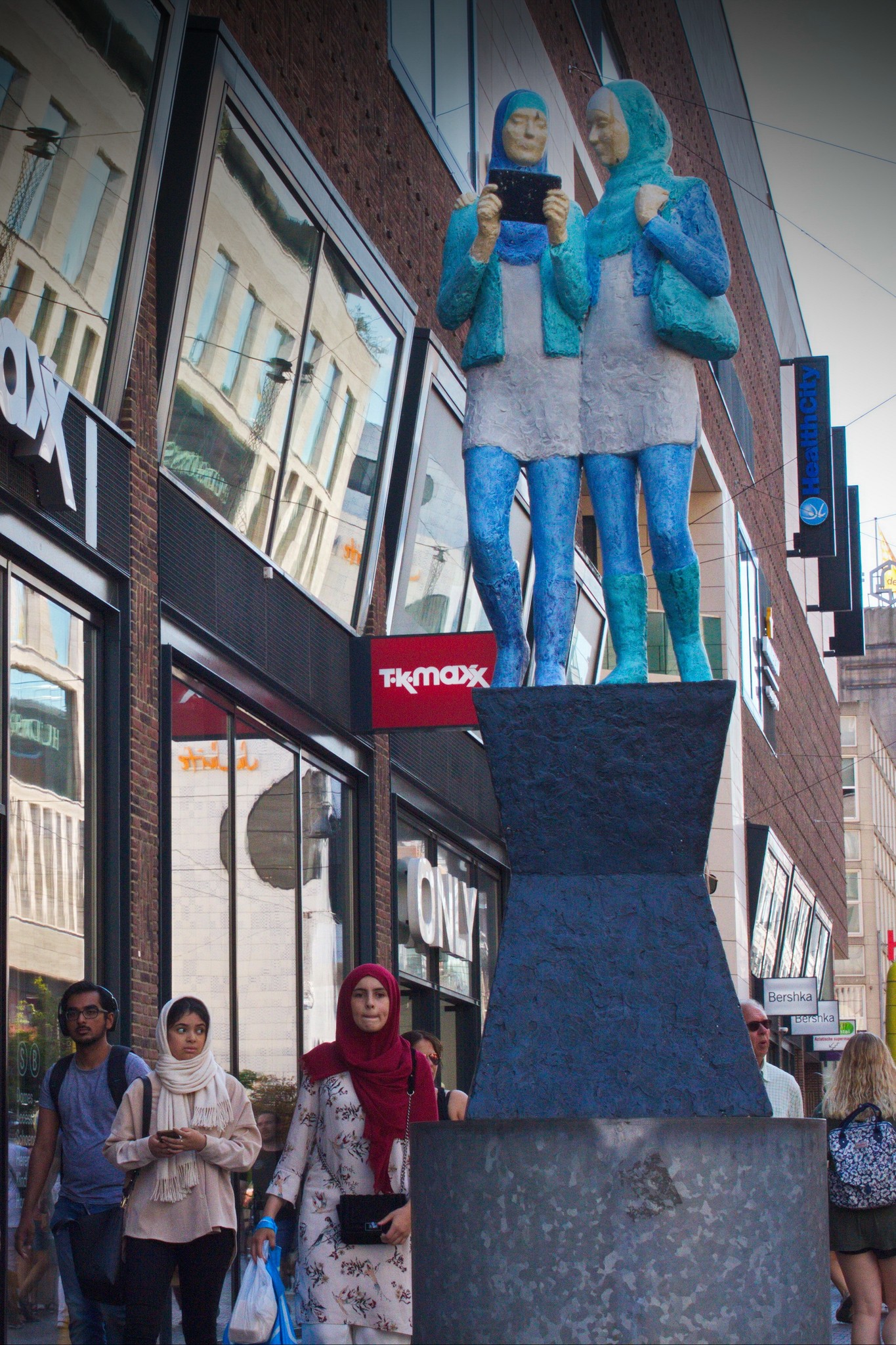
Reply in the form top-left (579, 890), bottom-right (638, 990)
top-left (156, 1130), bottom-right (180, 1142)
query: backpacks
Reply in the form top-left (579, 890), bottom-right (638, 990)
top-left (825, 1104), bottom-right (896, 1211)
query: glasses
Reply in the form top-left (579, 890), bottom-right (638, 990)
top-left (747, 1019), bottom-right (773, 1033)
top-left (423, 1053), bottom-right (441, 1065)
top-left (65, 1005), bottom-right (108, 1021)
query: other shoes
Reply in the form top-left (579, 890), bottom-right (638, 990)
top-left (57, 1321), bottom-right (69, 1329)
top-left (835, 1294), bottom-right (855, 1323)
top-left (880, 1303), bottom-right (889, 1315)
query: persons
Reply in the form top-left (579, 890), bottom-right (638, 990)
top-left (440, 79), bottom-right (739, 685)
top-left (102, 995), bottom-right (261, 1345)
top-left (796, 1032), bottom-right (896, 1345)
top-left (247, 963), bottom-right (438, 1345)
top-left (736, 996), bottom-right (803, 1118)
top-left (398, 1031), bottom-right (468, 1122)
top-left (6, 1124), bottom-right (72, 1327)
top-left (15, 979), bottom-right (154, 1345)
top-left (250, 1110), bottom-right (287, 1295)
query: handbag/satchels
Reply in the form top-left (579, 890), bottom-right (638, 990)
top-left (336, 1192), bottom-right (408, 1244)
top-left (122, 1167), bottom-right (138, 1195)
top-left (220, 1216), bottom-right (298, 1345)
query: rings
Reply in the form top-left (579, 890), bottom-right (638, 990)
top-left (180, 1139), bottom-right (184, 1145)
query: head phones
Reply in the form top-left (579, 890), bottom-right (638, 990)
top-left (56, 985), bottom-right (119, 1036)
